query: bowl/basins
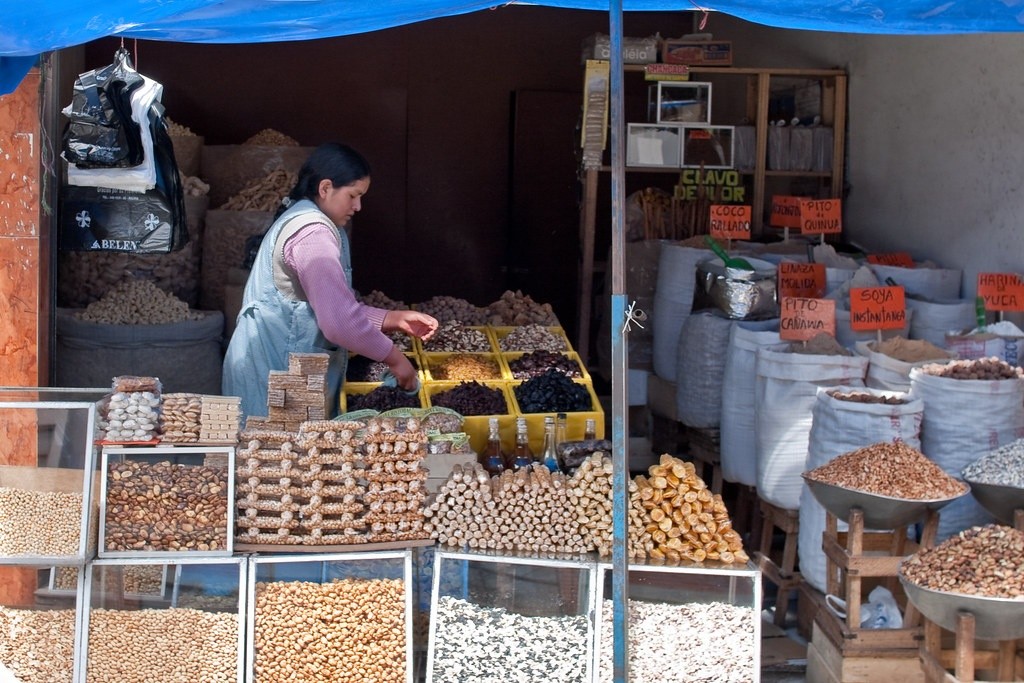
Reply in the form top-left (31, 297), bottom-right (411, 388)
top-left (960, 472), bottom-right (1024, 527)
top-left (897, 566), bottom-right (1024, 640)
top-left (802, 471), bottom-right (970, 529)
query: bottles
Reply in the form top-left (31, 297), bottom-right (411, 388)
top-left (584, 419), bottom-right (597, 440)
top-left (479, 413), bottom-right (567, 477)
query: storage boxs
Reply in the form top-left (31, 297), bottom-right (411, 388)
top-left (661, 42), bottom-right (732, 66)
top-left (648, 80), bottom-right (711, 125)
top-left (1, 306), bottom-right (765, 683)
top-left (624, 122), bottom-right (682, 168)
top-left (680, 124), bottom-right (736, 170)
top-left (580, 42), bottom-right (658, 66)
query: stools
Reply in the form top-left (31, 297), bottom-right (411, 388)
top-left (651, 409), bottom-right (1024, 683)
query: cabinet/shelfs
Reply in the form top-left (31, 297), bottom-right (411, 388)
top-left (576, 64), bottom-right (847, 369)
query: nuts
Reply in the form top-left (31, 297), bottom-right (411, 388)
top-left (71, 280), bottom-right (207, 325)
top-left (0, 399), bottom-right (408, 683)
top-left (804, 440), bottom-right (966, 500)
top-left (899, 521), bottom-right (1024, 602)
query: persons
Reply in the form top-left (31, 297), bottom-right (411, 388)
top-left (221, 145), bottom-right (440, 430)
